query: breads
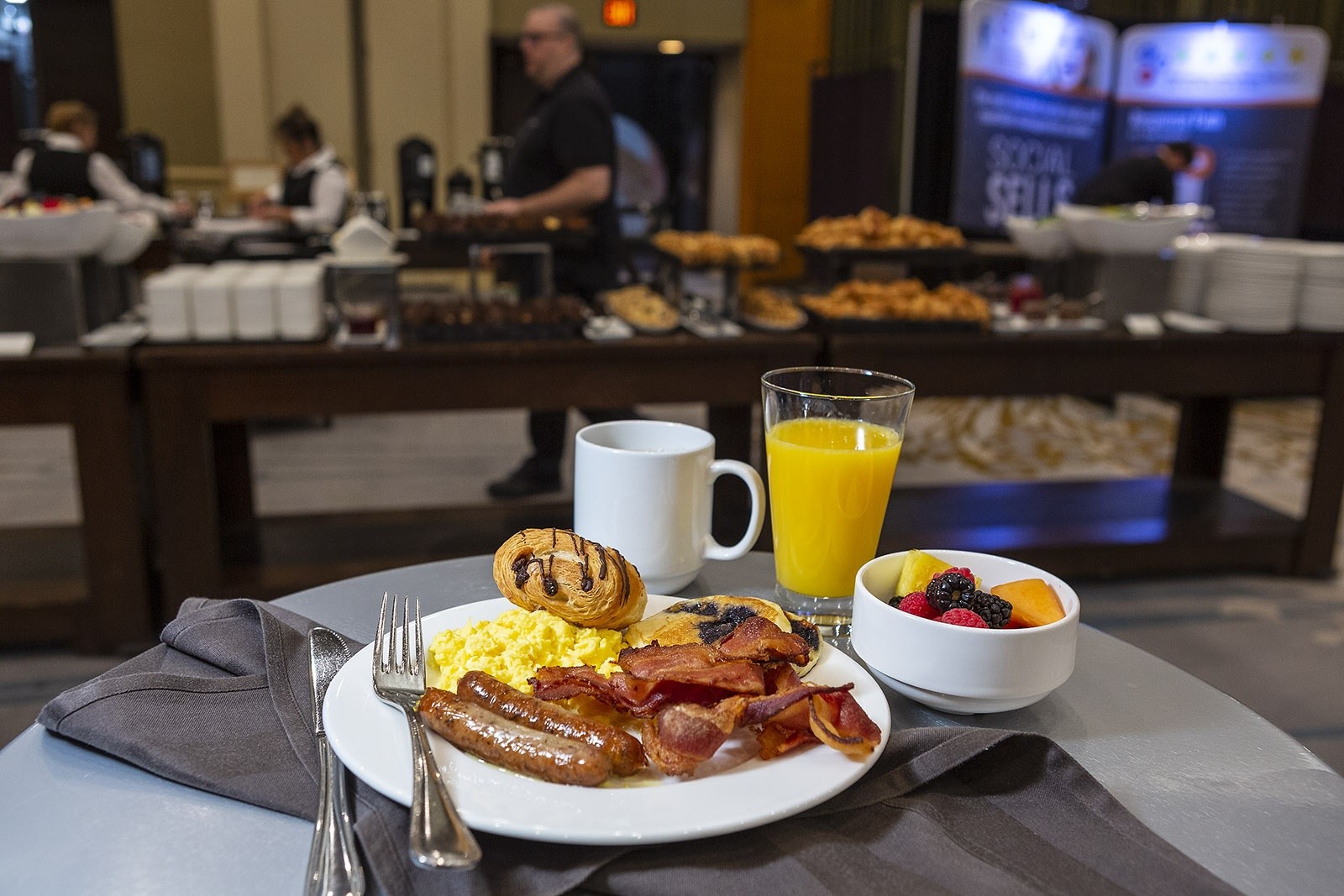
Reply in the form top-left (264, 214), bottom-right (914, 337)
top-left (605, 204), bottom-right (990, 329)
top-left (492, 528), bottom-right (648, 630)
top-left (626, 595), bottom-right (825, 678)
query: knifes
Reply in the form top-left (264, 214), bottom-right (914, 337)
top-left (304, 627), bottom-right (366, 896)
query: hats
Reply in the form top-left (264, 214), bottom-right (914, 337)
top-left (1165, 140), bottom-right (1191, 162)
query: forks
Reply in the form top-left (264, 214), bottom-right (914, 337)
top-left (374, 591), bottom-right (482, 872)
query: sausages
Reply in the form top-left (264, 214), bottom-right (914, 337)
top-left (416, 671), bottom-right (647, 787)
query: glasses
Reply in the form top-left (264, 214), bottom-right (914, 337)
top-left (522, 32), bottom-right (559, 44)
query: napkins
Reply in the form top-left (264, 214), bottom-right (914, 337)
top-left (38, 600), bottom-right (1237, 896)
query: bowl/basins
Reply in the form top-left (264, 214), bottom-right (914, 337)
top-left (1, 202), bottom-right (115, 260)
top-left (1054, 203), bottom-right (1200, 256)
top-left (851, 549), bottom-right (1080, 716)
top-left (1005, 215), bottom-right (1075, 258)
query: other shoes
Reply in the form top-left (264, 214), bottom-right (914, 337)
top-left (488, 458), bottom-right (564, 498)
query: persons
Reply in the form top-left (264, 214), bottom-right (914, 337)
top-left (1089, 144), bottom-right (1190, 206)
top-left (16, 102), bottom-right (193, 224)
top-left (614, 112), bottom-right (668, 242)
top-left (480, 2), bottom-right (641, 495)
top-left (247, 108), bottom-right (351, 237)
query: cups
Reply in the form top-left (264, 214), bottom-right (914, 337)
top-left (573, 420), bottom-right (767, 594)
top-left (761, 367), bottom-right (916, 637)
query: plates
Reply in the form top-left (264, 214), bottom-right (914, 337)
top-left (79, 255), bottom-right (324, 348)
top-left (322, 595), bottom-right (892, 845)
top-left (993, 305), bottom-right (1101, 335)
top-left (1160, 232), bottom-right (1344, 333)
top-left (677, 315), bottom-right (744, 340)
top-left (736, 305), bottom-right (807, 334)
top-left (0, 331), bottom-right (36, 356)
top-left (579, 315), bottom-right (634, 343)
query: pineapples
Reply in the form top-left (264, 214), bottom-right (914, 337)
top-left (894, 549), bottom-right (982, 597)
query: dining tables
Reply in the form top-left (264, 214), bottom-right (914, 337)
top-left (0, 548), bottom-right (1343, 896)
